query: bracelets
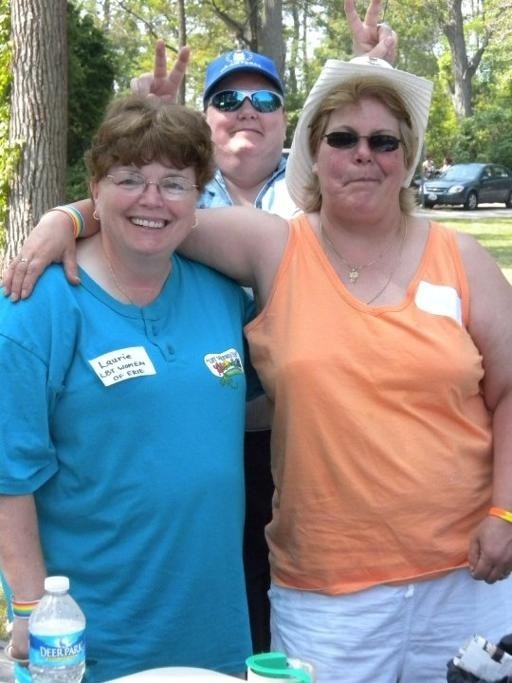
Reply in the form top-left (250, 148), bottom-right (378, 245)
top-left (50, 203), bottom-right (83, 236)
top-left (10, 598), bottom-right (39, 620)
top-left (486, 505), bottom-right (511, 522)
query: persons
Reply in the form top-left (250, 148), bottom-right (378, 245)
top-left (2, 56), bottom-right (511, 683)
top-left (1, 91), bottom-right (270, 683)
top-left (421, 153), bottom-right (434, 173)
top-left (131, 0), bottom-right (398, 444)
top-left (438, 157), bottom-right (453, 171)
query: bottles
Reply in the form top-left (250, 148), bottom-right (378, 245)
top-left (22, 573), bottom-right (89, 683)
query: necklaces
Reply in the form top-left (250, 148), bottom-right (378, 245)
top-left (368, 205), bottom-right (410, 301)
top-left (320, 228), bottom-right (397, 281)
top-left (102, 247), bottom-right (175, 310)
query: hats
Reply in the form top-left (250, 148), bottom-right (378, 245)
top-left (286, 56), bottom-right (433, 214)
top-left (204, 48), bottom-right (285, 108)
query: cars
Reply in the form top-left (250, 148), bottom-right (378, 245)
top-left (415, 159), bottom-right (512, 209)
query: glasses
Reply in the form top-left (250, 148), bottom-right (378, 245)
top-left (320, 131), bottom-right (407, 152)
top-left (104, 169), bottom-right (201, 203)
top-left (202, 88), bottom-right (285, 114)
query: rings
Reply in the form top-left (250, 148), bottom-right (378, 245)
top-left (19, 257), bottom-right (29, 262)
